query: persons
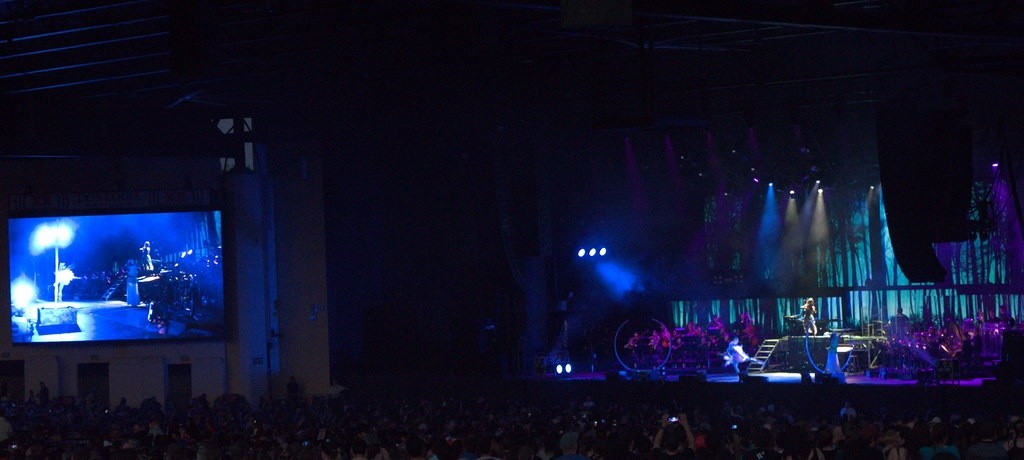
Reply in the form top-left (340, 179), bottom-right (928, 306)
top-left (628, 309), bottom-right (760, 382)
top-left (891, 304), bottom-right (1024, 379)
top-left (139, 241), bottom-right (153, 278)
top-left (0, 370), bottom-right (1024, 460)
top-left (804, 298), bottom-right (818, 336)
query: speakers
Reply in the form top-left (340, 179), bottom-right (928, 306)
top-left (877, 107), bottom-right (946, 283)
top-left (493, 128), bottom-right (535, 293)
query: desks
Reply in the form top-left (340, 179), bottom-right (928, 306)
top-left (844, 335), bottom-right (887, 373)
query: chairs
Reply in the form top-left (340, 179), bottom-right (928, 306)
top-left (935, 347), bottom-right (970, 385)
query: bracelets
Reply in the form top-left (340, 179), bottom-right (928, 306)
top-left (659, 427), bottom-right (665, 430)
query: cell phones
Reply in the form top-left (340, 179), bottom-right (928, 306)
top-left (667, 416), bottom-right (680, 423)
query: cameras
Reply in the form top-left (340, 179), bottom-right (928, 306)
top-left (731, 425), bottom-right (738, 430)
